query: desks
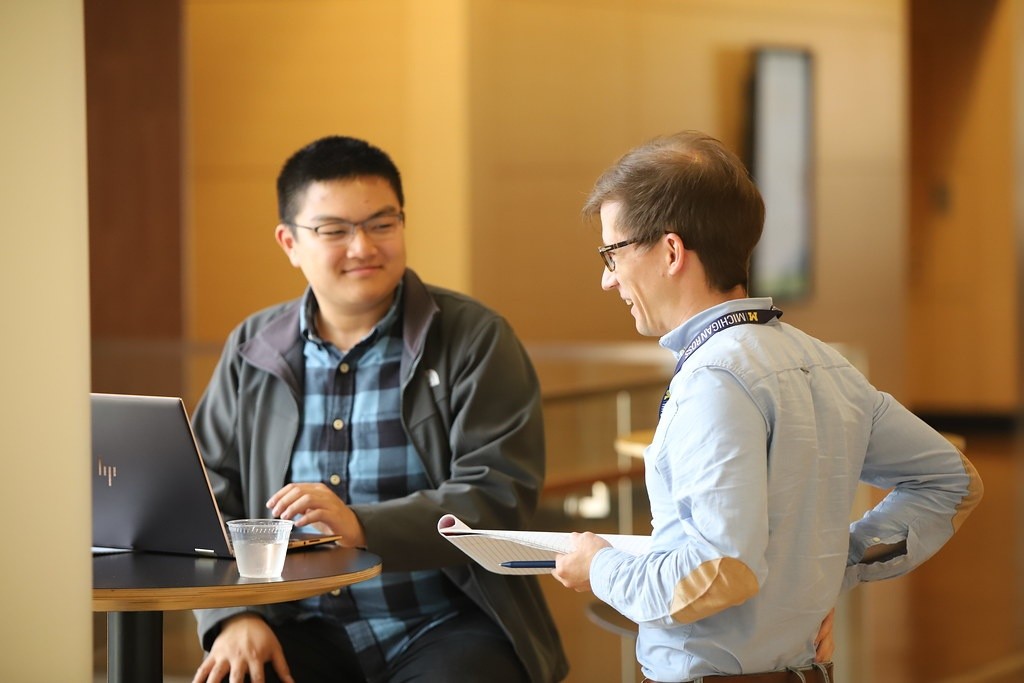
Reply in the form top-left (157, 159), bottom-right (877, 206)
top-left (528, 340), bottom-right (678, 537)
top-left (91, 543), bottom-right (381, 683)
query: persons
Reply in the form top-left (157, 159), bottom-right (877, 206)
top-left (180, 134), bottom-right (578, 681)
top-left (554, 130), bottom-right (986, 682)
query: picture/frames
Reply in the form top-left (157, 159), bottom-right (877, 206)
top-left (745, 47), bottom-right (818, 309)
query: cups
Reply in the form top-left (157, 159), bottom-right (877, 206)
top-left (226, 519), bottom-right (294, 577)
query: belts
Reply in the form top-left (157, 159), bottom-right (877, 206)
top-left (641, 663), bottom-right (834, 683)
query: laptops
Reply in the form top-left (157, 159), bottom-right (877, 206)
top-left (92, 394), bottom-right (344, 562)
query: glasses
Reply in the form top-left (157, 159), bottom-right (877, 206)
top-left (281, 213), bottom-right (405, 241)
top-left (597, 230), bottom-right (678, 273)
